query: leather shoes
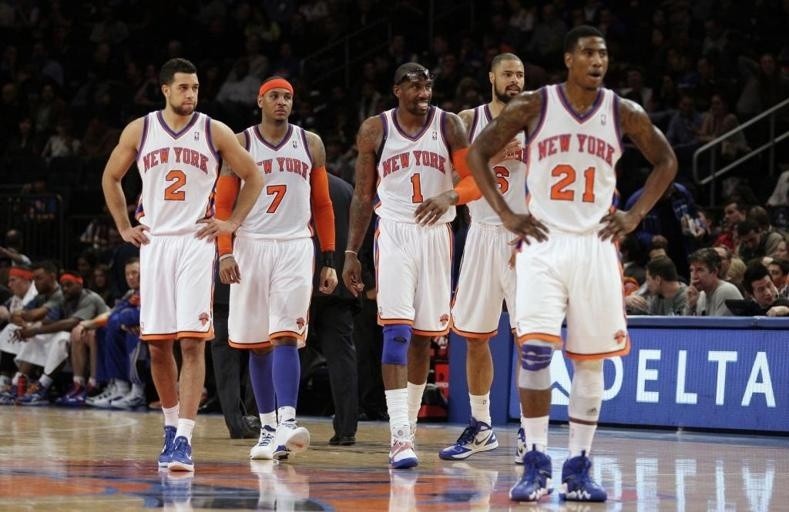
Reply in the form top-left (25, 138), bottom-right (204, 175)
top-left (328, 434), bottom-right (357, 444)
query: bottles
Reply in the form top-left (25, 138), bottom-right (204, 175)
top-left (17, 375), bottom-right (26, 397)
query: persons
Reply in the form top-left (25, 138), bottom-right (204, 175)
top-left (103, 58), bottom-right (265, 473)
top-left (54, 259), bottom-right (140, 406)
top-left (467, 25), bottom-right (678, 503)
top-left (1, 261), bottom-right (61, 403)
top-left (86, 292), bottom-right (161, 410)
top-left (211, 260), bottom-right (260, 436)
top-left (306, 167), bottom-right (358, 446)
top-left (439, 52), bottom-right (544, 463)
top-left (9, 272), bottom-right (114, 405)
top-left (0, 0), bottom-right (788, 330)
top-left (341, 64), bottom-right (481, 469)
top-left (217, 75), bottom-right (338, 458)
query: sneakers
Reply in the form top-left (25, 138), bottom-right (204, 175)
top-left (250, 419), bottom-right (310, 459)
top-left (158, 426), bottom-right (193, 472)
top-left (559, 456), bottom-right (607, 501)
top-left (439, 417), bottom-right (498, 459)
top-left (389, 421), bottom-right (418, 467)
top-left (0, 382), bottom-right (208, 408)
top-left (509, 431), bottom-right (554, 500)
